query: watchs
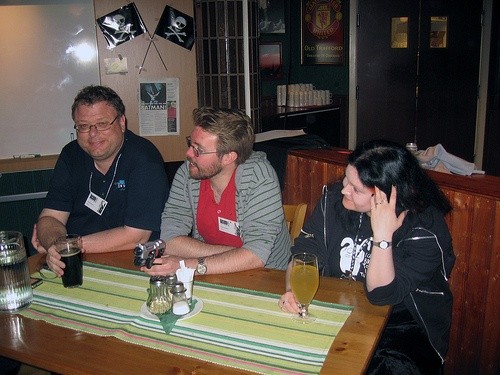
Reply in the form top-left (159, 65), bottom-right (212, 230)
top-left (196, 257), bottom-right (207, 275)
top-left (372, 239), bottom-right (393, 250)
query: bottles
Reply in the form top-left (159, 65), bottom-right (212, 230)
top-left (146, 274), bottom-right (190, 316)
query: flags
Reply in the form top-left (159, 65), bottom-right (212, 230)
top-left (97, 3), bottom-right (147, 49)
top-left (155, 5), bottom-right (194, 51)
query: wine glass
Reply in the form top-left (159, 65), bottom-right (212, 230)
top-left (291, 253), bottom-right (319, 323)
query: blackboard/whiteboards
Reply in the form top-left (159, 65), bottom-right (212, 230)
top-left (0, 0), bottom-right (101, 159)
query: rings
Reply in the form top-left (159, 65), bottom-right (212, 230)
top-left (282, 301), bottom-right (287, 304)
top-left (280, 305), bottom-right (283, 310)
top-left (376, 202), bottom-right (382, 205)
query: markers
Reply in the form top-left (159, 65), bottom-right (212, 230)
top-left (21, 155), bottom-right (40, 158)
top-left (13, 155), bottom-right (21, 159)
top-left (70, 133), bottom-right (74, 141)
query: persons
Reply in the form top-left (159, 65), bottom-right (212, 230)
top-left (277, 140), bottom-right (456, 375)
top-left (30, 85), bottom-right (169, 278)
top-left (139, 108), bottom-right (292, 278)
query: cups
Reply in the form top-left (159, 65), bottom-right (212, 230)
top-left (277, 81), bottom-right (333, 108)
top-left (406, 143), bottom-right (418, 155)
top-left (53, 234), bottom-right (84, 289)
top-left (177, 269), bottom-right (195, 299)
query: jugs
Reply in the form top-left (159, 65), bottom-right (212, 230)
top-left (0, 230), bottom-right (33, 314)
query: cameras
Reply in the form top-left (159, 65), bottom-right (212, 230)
top-left (133, 238), bottom-right (166, 269)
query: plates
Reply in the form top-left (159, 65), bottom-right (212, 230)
top-left (141, 295), bottom-right (204, 322)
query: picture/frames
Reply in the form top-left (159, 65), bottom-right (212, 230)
top-left (259, 40), bottom-right (283, 82)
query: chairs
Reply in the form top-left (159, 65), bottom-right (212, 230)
top-left (284, 201), bottom-right (306, 245)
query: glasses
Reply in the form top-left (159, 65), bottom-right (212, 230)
top-left (74, 113), bottom-right (118, 133)
top-left (185, 136), bottom-right (240, 158)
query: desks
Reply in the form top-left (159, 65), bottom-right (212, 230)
top-left (0, 246), bottom-right (394, 375)
top-left (258, 95), bottom-right (346, 153)
